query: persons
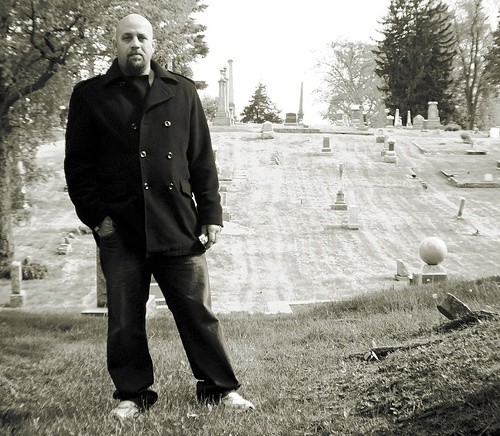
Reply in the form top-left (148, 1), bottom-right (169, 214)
top-left (63, 13), bottom-right (256, 421)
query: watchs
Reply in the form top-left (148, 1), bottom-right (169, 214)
top-left (93, 220), bottom-right (103, 232)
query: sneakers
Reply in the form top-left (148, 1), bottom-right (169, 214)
top-left (219, 390), bottom-right (255, 414)
top-left (110, 400), bottom-right (139, 422)
